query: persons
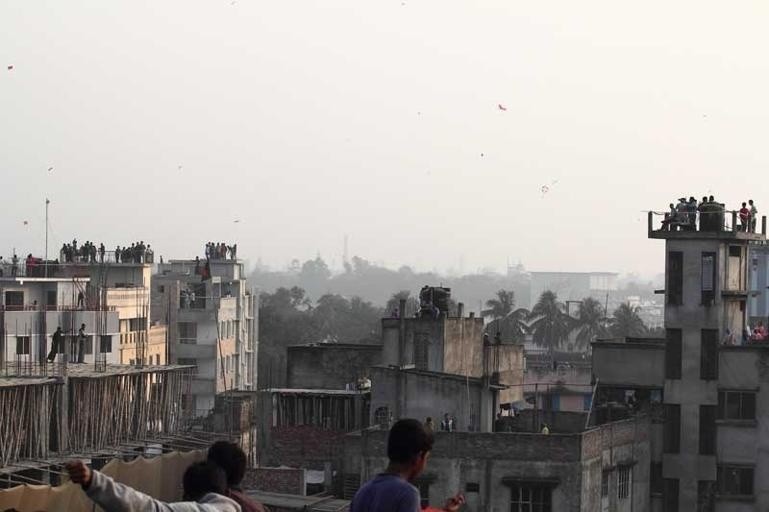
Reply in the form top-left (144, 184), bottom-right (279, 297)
top-left (78, 323), bottom-right (88, 364)
top-left (182, 289), bottom-right (196, 310)
top-left (0, 253), bottom-right (34, 278)
top-left (47, 326), bottom-right (64, 362)
top-left (541, 423), bottom-right (549, 434)
top-left (740, 201), bottom-right (752, 232)
top-left (192, 256), bottom-right (200, 262)
top-left (483, 333), bottom-right (492, 344)
top-left (657, 196), bottom-right (715, 232)
top-left (424, 417), bottom-right (435, 431)
top-left (722, 328), bottom-right (736, 345)
top-left (748, 200), bottom-right (758, 233)
top-left (32, 301), bottom-right (38, 310)
top-left (440, 413), bottom-right (455, 433)
top-left (392, 308), bottom-right (398, 317)
top-left (750, 322), bottom-right (766, 341)
top-left (350, 417), bottom-right (466, 512)
top-left (63, 458), bottom-right (243, 512)
top-left (494, 333), bottom-right (502, 345)
top-left (61, 238), bottom-right (152, 263)
top-left (205, 242), bottom-right (237, 261)
top-left (210, 439), bottom-right (267, 511)
